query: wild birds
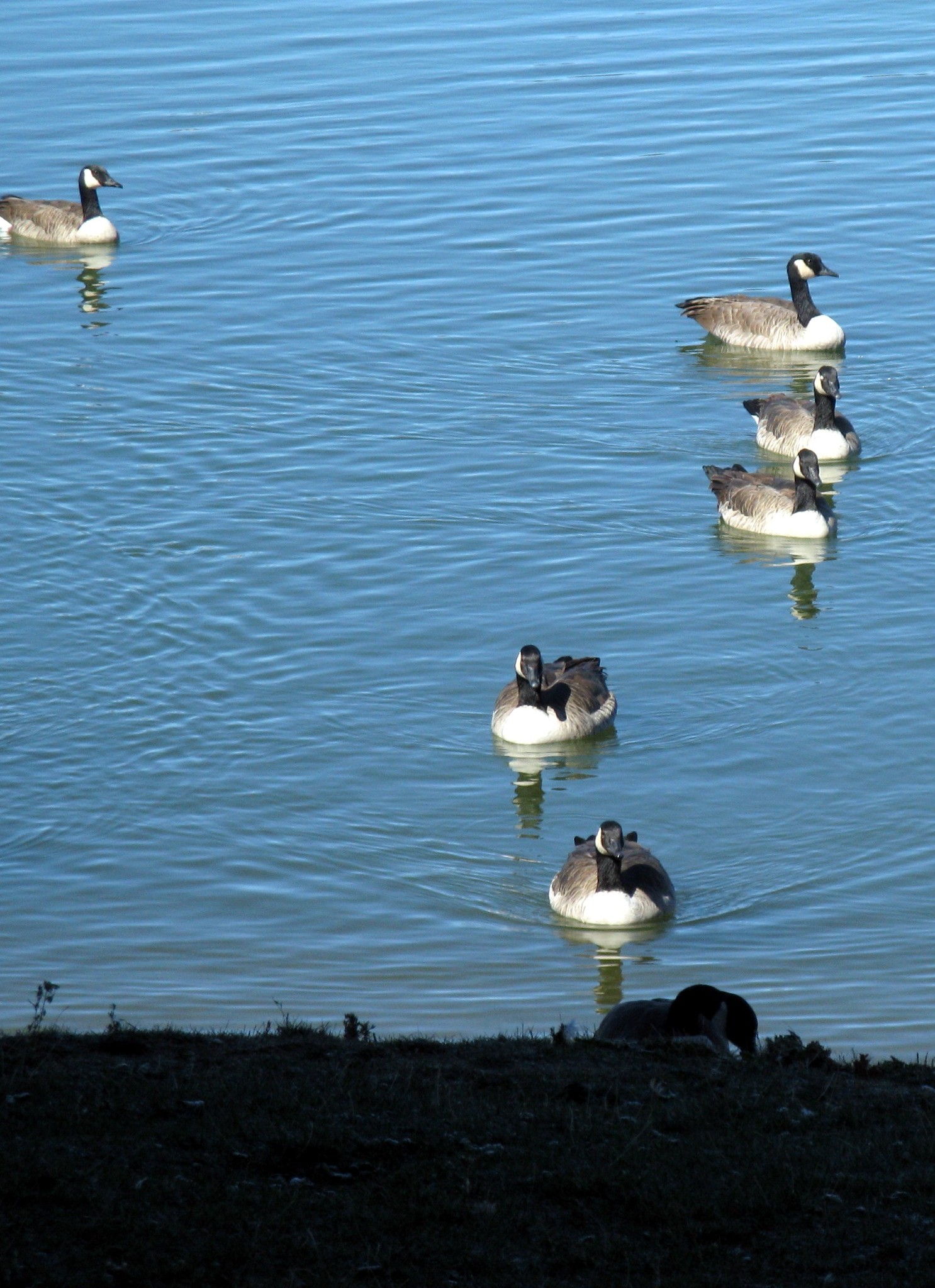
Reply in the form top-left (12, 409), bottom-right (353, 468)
top-left (549, 908), bottom-right (676, 1015)
top-left (680, 331), bottom-right (845, 400)
top-left (492, 723), bottom-right (618, 817)
top-left (492, 644), bottom-right (618, 745)
top-left (674, 252), bottom-right (846, 351)
top-left (0, 164), bottom-right (123, 244)
top-left (702, 449), bottom-right (837, 538)
top-left (712, 514), bottom-right (838, 620)
top-left (548, 820), bottom-right (677, 926)
top-left (743, 365), bottom-right (862, 461)
